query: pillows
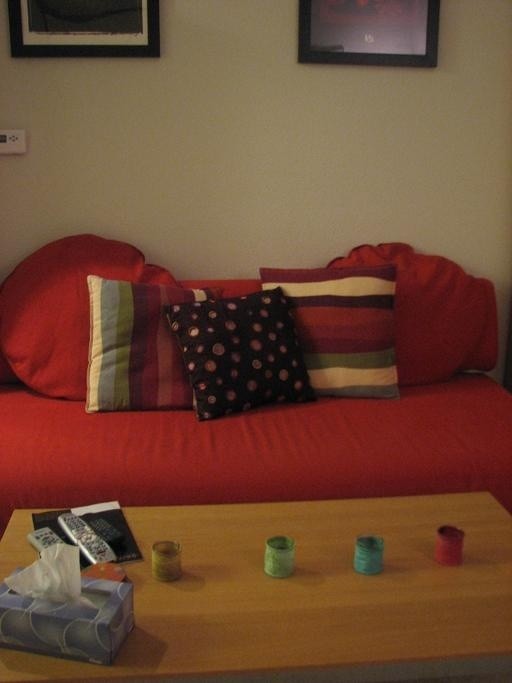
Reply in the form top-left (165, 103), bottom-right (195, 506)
top-left (256, 265), bottom-right (400, 399)
top-left (163, 287), bottom-right (316, 420)
top-left (85, 275), bottom-right (208, 411)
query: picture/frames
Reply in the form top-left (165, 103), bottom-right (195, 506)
top-left (8, 0), bottom-right (160, 58)
top-left (298, 0), bottom-right (440, 68)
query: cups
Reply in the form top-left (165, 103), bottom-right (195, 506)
top-left (264, 535), bottom-right (295, 578)
top-left (352, 535), bottom-right (385, 575)
top-left (436, 524), bottom-right (465, 567)
top-left (151, 540), bottom-right (183, 582)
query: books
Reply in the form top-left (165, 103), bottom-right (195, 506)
top-left (32, 499), bottom-right (144, 570)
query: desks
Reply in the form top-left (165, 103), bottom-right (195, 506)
top-left (0, 492), bottom-right (512, 683)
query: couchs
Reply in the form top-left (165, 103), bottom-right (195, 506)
top-left (0, 278), bottom-right (512, 537)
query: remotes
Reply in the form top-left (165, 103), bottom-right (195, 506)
top-left (55, 511), bottom-right (121, 564)
top-left (28, 526), bottom-right (63, 555)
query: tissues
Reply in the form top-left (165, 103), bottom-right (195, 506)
top-left (0, 540), bottom-right (137, 664)
